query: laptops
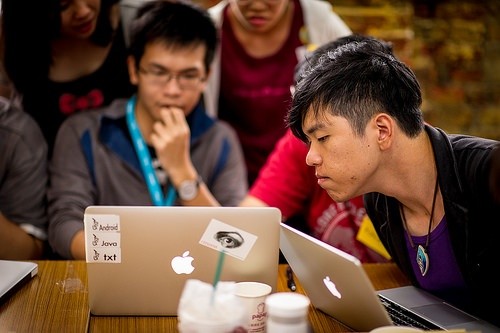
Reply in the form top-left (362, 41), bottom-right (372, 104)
top-left (280, 223), bottom-right (500, 333)
top-left (84, 206), bottom-right (282, 316)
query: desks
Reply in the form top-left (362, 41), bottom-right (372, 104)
top-left (0, 260), bottom-right (412, 333)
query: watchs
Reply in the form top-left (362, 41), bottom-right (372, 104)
top-left (177, 174), bottom-right (203, 201)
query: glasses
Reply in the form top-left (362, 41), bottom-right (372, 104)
top-left (138, 66), bottom-right (206, 89)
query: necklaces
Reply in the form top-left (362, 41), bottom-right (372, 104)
top-left (399, 175), bottom-right (439, 276)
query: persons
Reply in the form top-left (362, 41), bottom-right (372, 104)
top-left (285, 40), bottom-right (500, 329)
top-left (0, 0), bottom-right (393, 263)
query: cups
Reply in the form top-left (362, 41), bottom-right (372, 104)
top-left (230, 282), bottom-right (272, 333)
top-left (265, 292), bottom-right (310, 333)
